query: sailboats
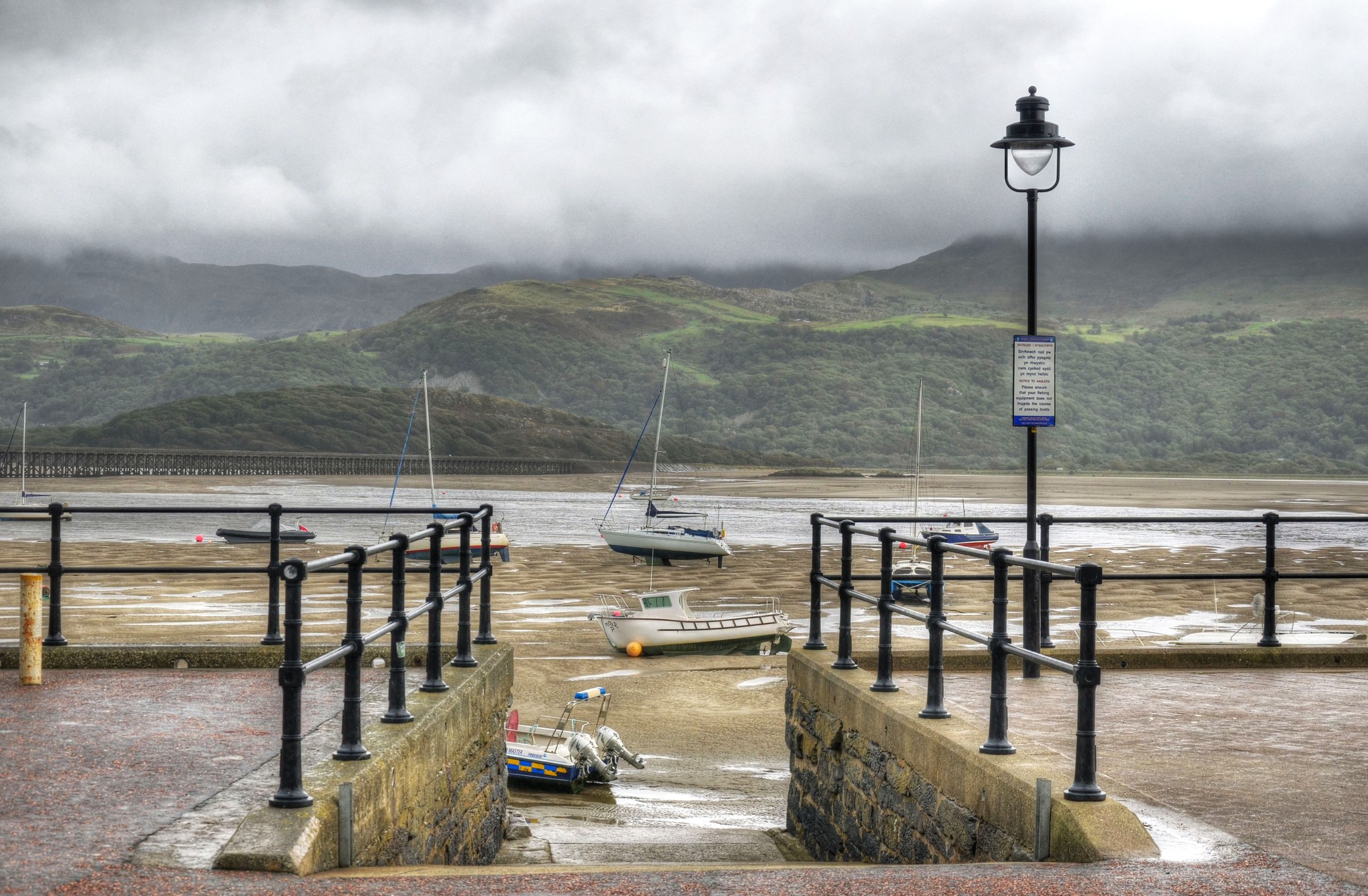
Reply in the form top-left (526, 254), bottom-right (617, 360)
top-left (891, 376), bottom-right (933, 600)
top-left (591, 348), bottom-right (734, 569)
top-left (369, 368), bottom-right (511, 566)
top-left (0, 398), bottom-right (74, 522)
top-left (628, 462), bottom-right (673, 500)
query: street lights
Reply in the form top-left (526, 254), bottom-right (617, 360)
top-left (986, 85), bottom-right (1075, 678)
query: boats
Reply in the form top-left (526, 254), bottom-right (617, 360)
top-left (585, 546), bottom-right (798, 658)
top-left (919, 499), bottom-right (999, 551)
top-left (1070, 580), bottom-right (1363, 646)
top-left (504, 685), bottom-right (647, 795)
top-left (214, 515), bottom-right (318, 544)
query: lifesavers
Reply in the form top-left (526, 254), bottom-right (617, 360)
top-left (723, 529), bottom-right (725, 537)
